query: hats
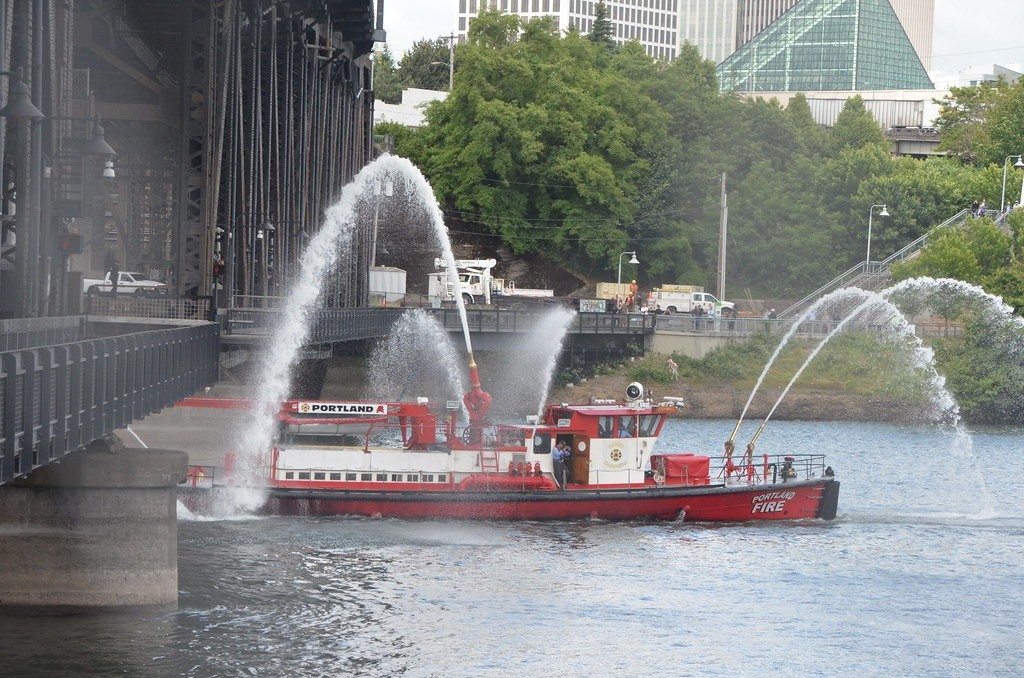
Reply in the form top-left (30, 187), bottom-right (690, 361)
top-left (559, 439), bottom-right (567, 445)
top-left (632, 279), bottom-right (637, 284)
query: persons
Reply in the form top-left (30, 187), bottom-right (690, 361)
top-left (971, 197), bottom-right (988, 218)
top-left (552, 440), bottom-right (571, 490)
top-left (762, 307), bottom-right (777, 332)
top-left (598, 418), bottom-right (634, 437)
top-left (610, 280), bottom-right (672, 315)
top-left (792, 309), bottom-right (840, 334)
top-left (727, 304), bottom-right (739, 330)
top-left (690, 304), bottom-right (715, 330)
top-left (108, 260), bottom-right (120, 298)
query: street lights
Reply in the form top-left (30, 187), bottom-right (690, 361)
top-left (1001, 154), bottom-right (1024, 213)
top-left (14, 116), bottom-right (118, 321)
top-left (227, 212), bottom-right (277, 337)
top-left (432, 61), bottom-right (455, 92)
top-left (617, 251), bottom-right (640, 310)
top-left (240, 218), bottom-right (310, 306)
top-left (867, 204), bottom-right (889, 273)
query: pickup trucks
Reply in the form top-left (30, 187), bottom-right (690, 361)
top-left (83, 271), bottom-right (167, 300)
top-left (648, 291), bottom-right (735, 318)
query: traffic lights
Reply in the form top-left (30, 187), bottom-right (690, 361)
top-left (60, 232), bottom-right (71, 252)
top-left (213, 264), bottom-right (224, 276)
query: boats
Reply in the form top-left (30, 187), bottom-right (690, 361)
top-left (177, 351), bottom-right (839, 524)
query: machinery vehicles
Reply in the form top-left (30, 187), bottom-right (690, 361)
top-left (434, 257), bottom-right (554, 309)
